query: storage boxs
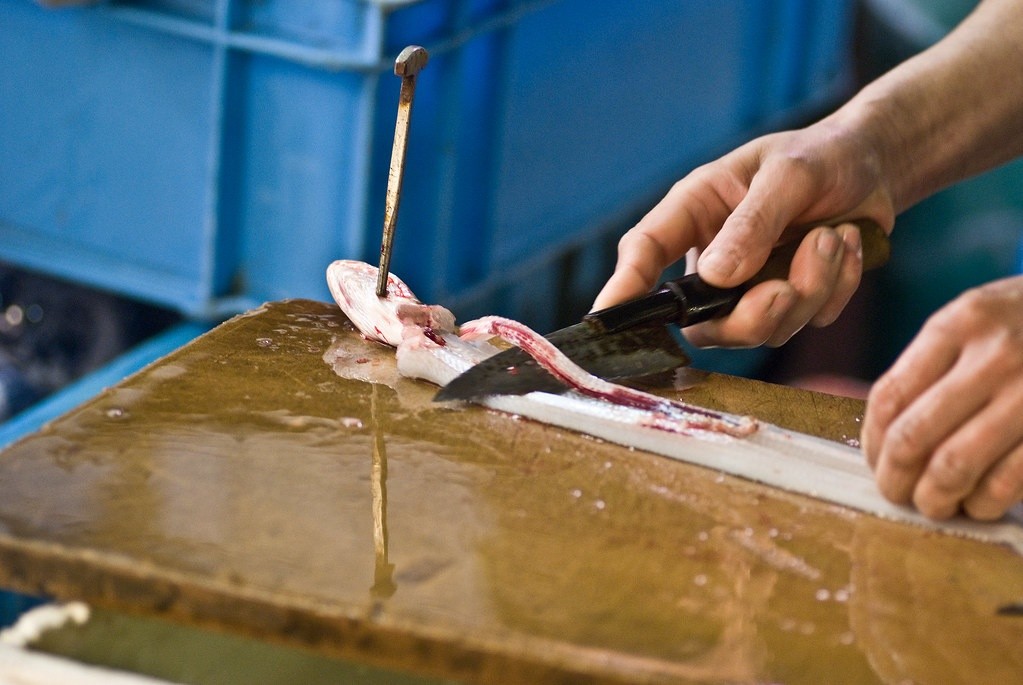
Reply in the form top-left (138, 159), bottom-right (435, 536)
top-left (0, 0), bottom-right (855, 323)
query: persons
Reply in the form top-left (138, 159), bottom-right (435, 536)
top-left (587, 0), bottom-right (1023, 521)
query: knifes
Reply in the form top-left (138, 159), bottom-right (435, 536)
top-left (432, 217), bottom-right (892, 403)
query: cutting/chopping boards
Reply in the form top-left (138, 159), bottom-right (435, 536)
top-left (0, 299), bottom-right (1023, 685)
